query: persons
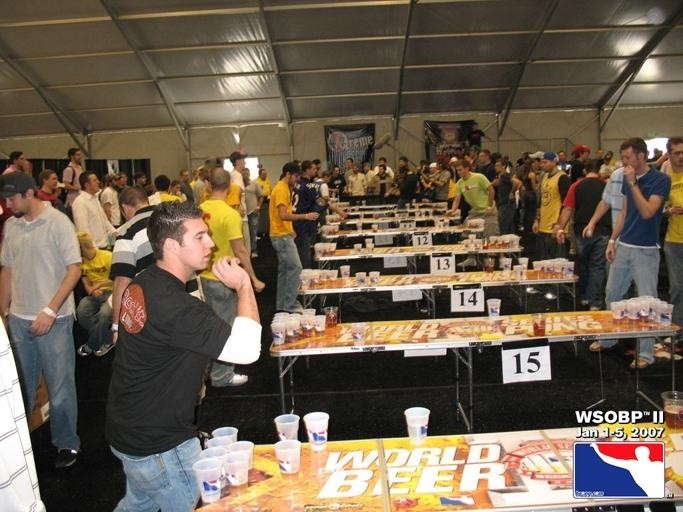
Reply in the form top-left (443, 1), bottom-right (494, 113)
top-left (102, 199), bottom-right (262, 511)
top-left (2, 136), bottom-right (683, 372)
top-left (191, 168), bottom-right (266, 389)
top-left (0, 315), bottom-right (49, 510)
top-left (1, 171), bottom-right (84, 469)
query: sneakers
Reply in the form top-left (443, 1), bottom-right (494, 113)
top-left (457, 258), bottom-right (477, 267)
top-left (526, 286), bottom-right (541, 294)
top-left (76, 342), bottom-right (92, 357)
top-left (55, 447), bottom-right (78, 468)
top-left (589, 340), bottom-right (603, 352)
top-left (663, 334), bottom-right (682, 345)
top-left (225, 372), bottom-right (248, 386)
top-left (629, 358), bottom-right (649, 369)
top-left (543, 292), bottom-right (557, 300)
top-left (92, 343), bottom-right (114, 357)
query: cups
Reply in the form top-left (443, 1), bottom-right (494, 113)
top-left (271, 294), bottom-right (370, 351)
top-left (660, 391), bottom-right (682, 438)
top-left (484, 256), bottom-right (575, 282)
top-left (298, 264), bottom-right (381, 288)
top-left (612, 295), bottom-right (675, 325)
top-left (316, 200), bottom-right (379, 256)
top-left (487, 299), bottom-right (501, 320)
top-left (193, 406), bottom-right (432, 503)
top-left (405, 198), bottom-right (521, 251)
top-left (529, 313), bottom-right (546, 336)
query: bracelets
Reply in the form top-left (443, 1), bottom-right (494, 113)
top-left (42, 305), bottom-right (58, 319)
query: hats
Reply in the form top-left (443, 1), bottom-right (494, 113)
top-left (527, 151), bottom-right (544, 159)
top-left (541, 151), bottom-right (559, 163)
top-left (0, 171), bottom-right (37, 197)
top-left (229, 152), bottom-right (247, 162)
top-left (104, 173), bottom-right (120, 181)
top-left (573, 144), bottom-right (590, 152)
top-left (282, 162), bottom-right (303, 175)
